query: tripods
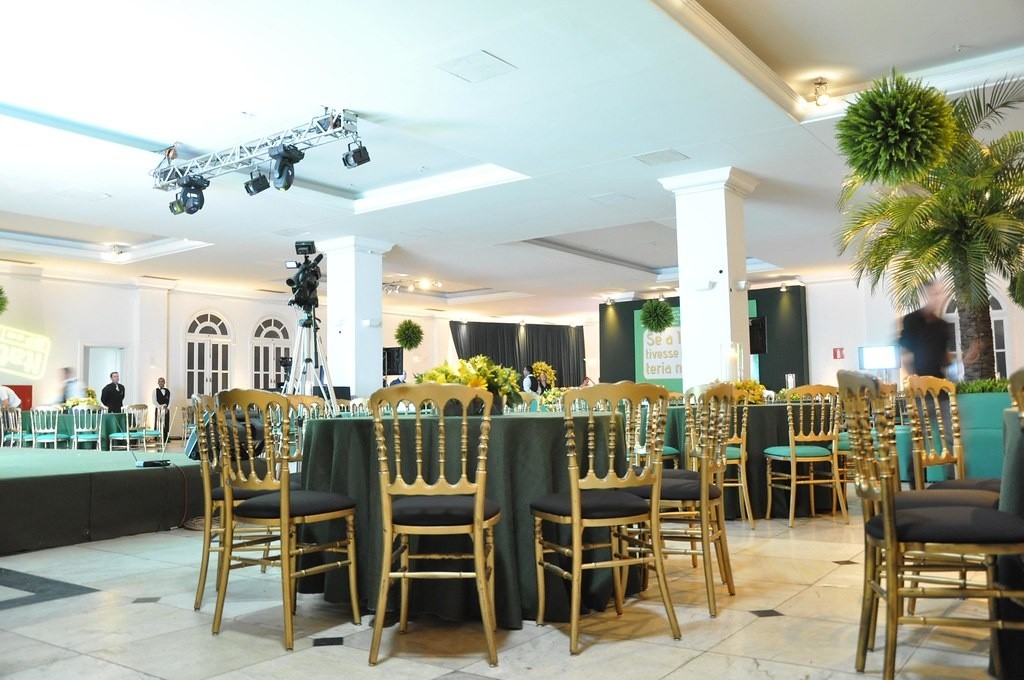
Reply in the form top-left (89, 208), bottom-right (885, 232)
top-left (281, 306), bottom-right (343, 418)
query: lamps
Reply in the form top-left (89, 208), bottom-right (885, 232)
top-left (813, 77), bottom-right (830, 106)
top-left (382, 277), bottom-right (442, 294)
top-left (605, 297), bottom-right (612, 306)
top-left (658, 293), bottom-right (665, 301)
top-left (779, 283), bottom-right (788, 292)
top-left (243, 145), bottom-right (308, 195)
top-left (341, 133), bottom-right (371, 170)
top-left (168, 174), bottom-right (211, 215)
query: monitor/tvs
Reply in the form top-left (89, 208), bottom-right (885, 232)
top-left (858, 345), bottom-right (901, 370)
top-left (749, 316), bottom-right (768, 354)
top-left (383, 347), bottom-right (403, 375)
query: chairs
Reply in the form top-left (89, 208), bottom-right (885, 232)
top-left (180, 382), bottom-right (1024, 680)
top-left (0, 403), bottom-right (167, 452)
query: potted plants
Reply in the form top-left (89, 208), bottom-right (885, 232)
top-left (832, 66), bottom-right (1023, 482)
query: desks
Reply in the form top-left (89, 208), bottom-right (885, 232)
top-left (639, 402), bottom-right (849, 520)
top-left (294, 411), bottom-right (647, 630)
top-left (20, 412), bottom-right (137, 452)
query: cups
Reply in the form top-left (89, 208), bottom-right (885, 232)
top-left (785, 373), bottom-right (796, 392)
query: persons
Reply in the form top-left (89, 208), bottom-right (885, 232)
top-left (579, 377), bottom-right (595, 387)
top-left (47, 366), bottom-right (88, 413)
top-left (390, 370), bottom-right (406, 386)
top-left (0, 386), bottom-right (23, 427)
top-left (102, 372), bottom-right (125, 413)
top-left (901, 282), bottom-right (979, 379)
top-left (540, 374), bottom-right (551, 395)
top-left (153, 377), bottom-right (171, 443)
top-left (522, 365), bottom-right (541, 395)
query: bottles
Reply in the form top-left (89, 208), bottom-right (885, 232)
top-left (837, 349), bottom-right (841, 358)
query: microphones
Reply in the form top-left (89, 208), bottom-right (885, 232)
top-left (313, 254), bottom-right (323, 268)
